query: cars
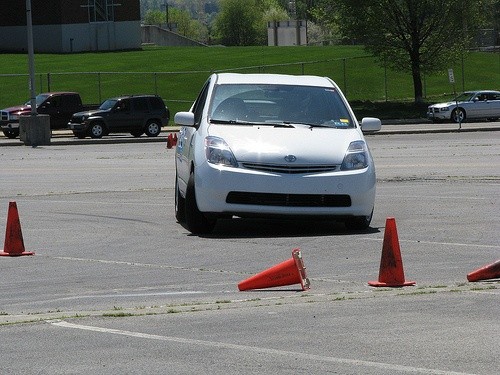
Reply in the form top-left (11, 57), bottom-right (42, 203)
top-left (427, 90), bottom-right (500, 124)
top-left (173, 72), bottom-right (382, 233)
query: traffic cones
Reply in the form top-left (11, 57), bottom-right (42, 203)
top-left (174, 132), bottom-right (178, 144)
top-left (167, 135), bottom-right (173, 149)
top-left (467, 259), bottom-right (500, 282)
top-left (237, 248), bottom-right (310, 291)
top-left (170, 133), bottom-right (173, 146)
top-left (0, 200), bottom-right (35, 257)
top-left (369, 218), bottom-right (416, 287)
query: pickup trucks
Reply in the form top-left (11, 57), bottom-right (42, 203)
top-left (0, 91), bottom-right (109, 139)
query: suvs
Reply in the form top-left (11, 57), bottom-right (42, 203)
top-left (68, 95), bottom-right (170, 139)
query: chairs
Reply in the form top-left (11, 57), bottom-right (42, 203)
top-left (220, 98), bottom-right (244, 118)
